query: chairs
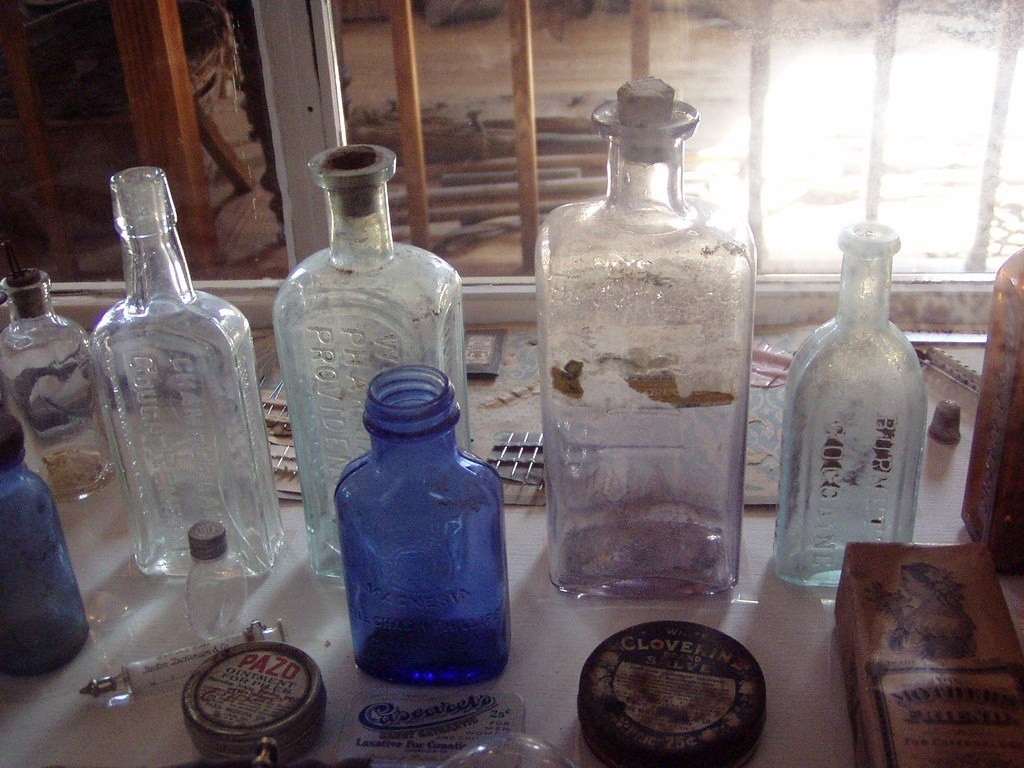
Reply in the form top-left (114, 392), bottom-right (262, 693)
top-left (0, 0), bottom-right (254, 253)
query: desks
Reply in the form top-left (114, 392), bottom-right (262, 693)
top-left (1, 326), bottom-right (1024, 768)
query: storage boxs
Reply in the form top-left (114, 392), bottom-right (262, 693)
top-left (836, 539), bottom-right (1024, 768)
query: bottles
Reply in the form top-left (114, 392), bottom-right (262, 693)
top-left (0, 412), bottom-right (85, 675)
top-left (88, 167), bottom-right (280, 580)
top-left (773, 221), bottom-right (927, 586)
top-left (961, 247), bottom-right (1024, 571)
top-left (334, 366), bottom-right (512, 685)
top-left (0, 266), bottom-right (114, 501)
top-left (535, 75), bottom-right (757, 600)
top-left (187, 519), bottom-right (247, 641)
top-left (274, 145), bottom-right (470, 579)
